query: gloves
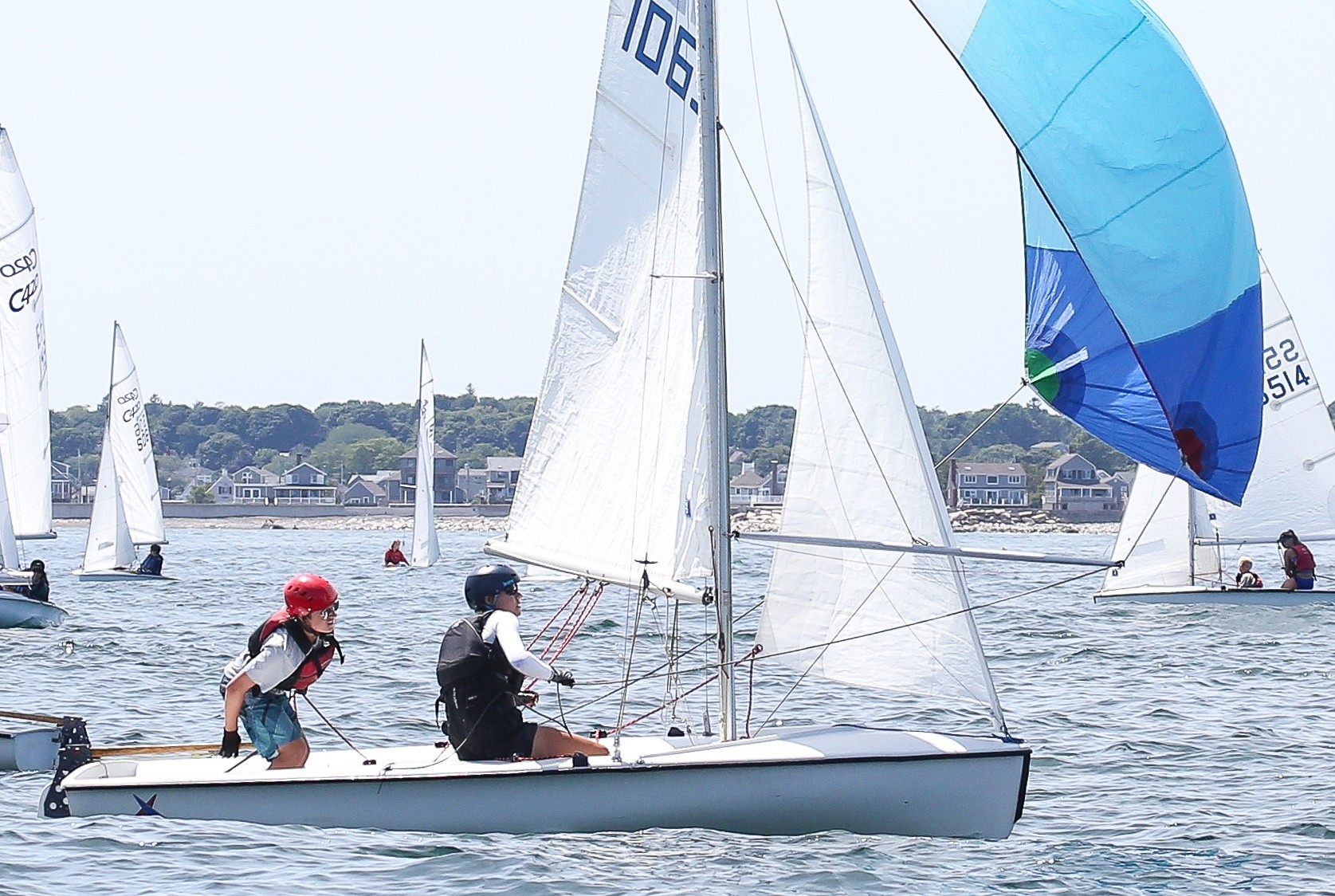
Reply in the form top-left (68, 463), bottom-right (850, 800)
top-left (553, 667), bottom-right (575, 687)
top-left (516, 690), bottom-right (540, 707)
top-left (218, 726), bottom-right (241, 759)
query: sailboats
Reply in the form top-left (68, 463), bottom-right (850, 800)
top-left (379, 336), bottom-right (444, 576)
top-left (1085, 236), bottom-right (1335, 603)
top-left (0, 121), bottom-right (74, 632)
top-left (43, 0), bottom-right (1269, 852)
top-left (70, 317), bottom-right (184, 583)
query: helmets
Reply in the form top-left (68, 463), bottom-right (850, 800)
top-left (464, 563), bottom-right (521, 609)
top-left (284, 571), bottom-right (338, 618)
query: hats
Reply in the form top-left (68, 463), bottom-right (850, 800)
top-left (1277, 532), bottom-right (1293, 541)
top-left (30, 560), bottom-right (45, 571)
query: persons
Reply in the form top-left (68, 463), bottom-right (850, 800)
top-left (384, 540), bottom-right (410, 568)
top-left (1236, 557), bottom-right (1264, 588)
top-left (216, 572), bottom-right (340, 770)
top-left (1277, 530), bottom-right (1316, 590)
top-left (6, 558), bottom-right (50, 603)
top-left (140, 544), bottom-right (163, 576)
top-left (434, 562), bottom-right (609, 761)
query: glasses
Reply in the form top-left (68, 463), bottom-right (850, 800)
top-left (320, 600), bottom-right (339, 620)
top-left (501, 584), bottom-right (518, 596)
